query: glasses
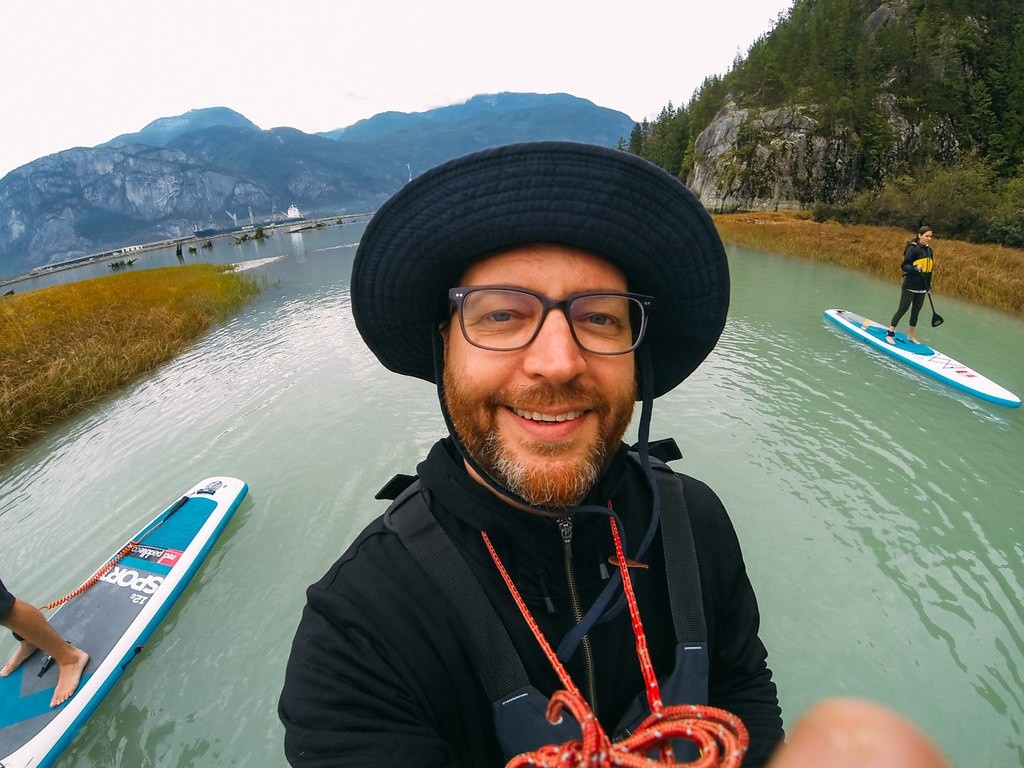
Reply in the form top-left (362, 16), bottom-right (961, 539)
top-left (446, 285), bottom-right (666, 356)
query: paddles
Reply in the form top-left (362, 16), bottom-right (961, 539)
top-left (920, 269), bottom-right (945, 328)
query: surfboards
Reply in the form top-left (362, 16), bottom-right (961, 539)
top-left (2, 472), bottom-right (250, 768)
top-left (824, 308), bottom-right (1022, 409)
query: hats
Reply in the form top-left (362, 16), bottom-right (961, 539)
top-left (350, 141), bottom-right (732, 402)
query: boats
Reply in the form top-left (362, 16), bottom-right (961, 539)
top-left (191, 207), bottom-right (317, 238)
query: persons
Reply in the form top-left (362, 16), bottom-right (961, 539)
top-left (276, 141), bottom-right (948, 768)
top-left (0, 578), bottom-right (90, 709)
top-left (885, 226), bottom-right (934, 345)
